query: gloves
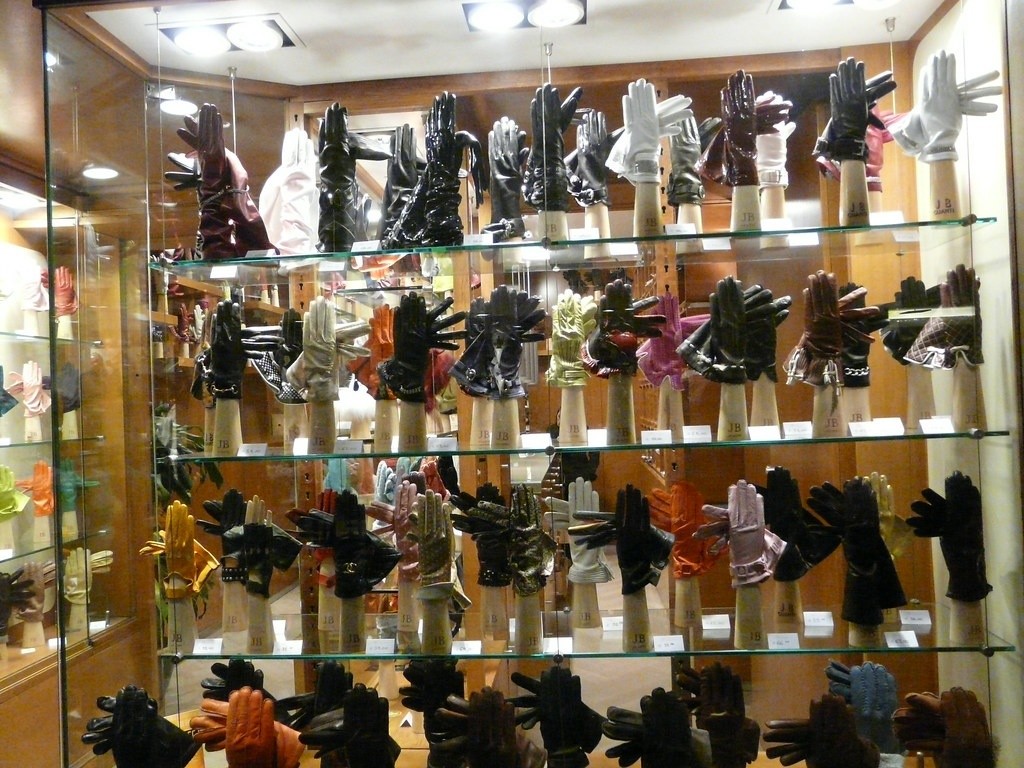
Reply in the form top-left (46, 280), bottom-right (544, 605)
top-left (0, 359), bottom-right (83, 418)
top-left (81, 656), bottom-right (1000, 768)
top-left (39, 264), bottom-right (83, 320)
top-left (0, 460), bottom-right (101, 523)
top-left (0, 545), bottom-right (114, 635)
top-left (166, 264), bottom-right (984, 403)
top-left (163, 50), bottom-right (1004, 262)
top-left (140, 455), bottom-right (993, 626)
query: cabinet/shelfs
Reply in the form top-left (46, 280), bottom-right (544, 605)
top-left (0, 131), bottom-right (158, 768)
top-left (32, 0), bottom-right (1024, 767)
top-left (145, 263), bottom-right (300, 644)
